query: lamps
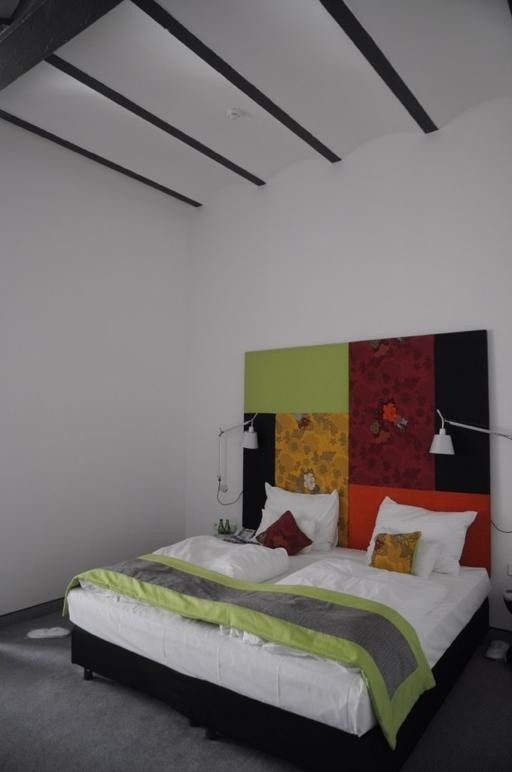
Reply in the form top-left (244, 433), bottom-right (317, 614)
top-left (429, 409), bottom-right (512, 455)
top-left (216, 412), bottom-right (258, 506)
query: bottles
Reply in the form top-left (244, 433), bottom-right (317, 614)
top-left (218, 519), bottom-right (233, 534)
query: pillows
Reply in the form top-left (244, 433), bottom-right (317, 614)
top-left (250, 482), bottom-right (478, 579)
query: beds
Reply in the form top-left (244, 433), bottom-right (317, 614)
top-left (62, 526), bottom-right (491, 771)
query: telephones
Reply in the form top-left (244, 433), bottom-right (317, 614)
top-left (234, 526), bottom-right (255, 543)
